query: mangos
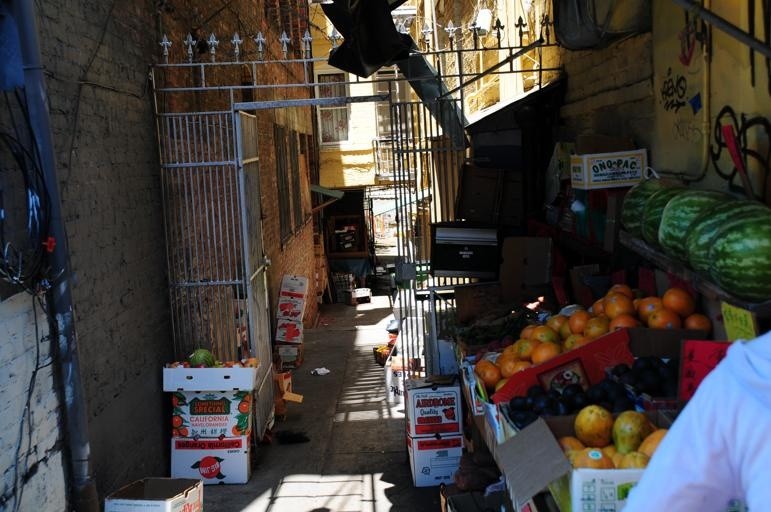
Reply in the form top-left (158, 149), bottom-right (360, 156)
top-left (560, 437), bottom-right (586, 464)
top-left (613, 410), bottom-right (651, 455)
top-left (574, 447), bottom-right (614, 468)
top-left (575, 405), bottom-right (613, 448)
top-left (638, 429), bottom-right (668, 456)
top-left (618, 451), bottom-right (651, 467)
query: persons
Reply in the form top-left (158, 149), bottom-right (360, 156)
top-left (619, 335), bottom-right (771, 511)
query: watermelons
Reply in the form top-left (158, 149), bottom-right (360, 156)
top-left (708, 209), bottom-right (770, 301)
top-left (683, 198), bottom-right (766, 281)
top-left (659, 190), bottom-right (743, 263)
top-left (641, 185), bottom-right (695, 252)
top-left (622, 176), bottom-right (688, 239)
top-left (189, 350), bottom-right (215, 368)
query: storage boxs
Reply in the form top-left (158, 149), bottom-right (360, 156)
top-left (404, 378), bottom-right (463, 438)
top-left (277, 296), bottom-right (307, 322)
top-left (173, 391), bottom-right (251, 437)
top-left (276, 344), bottom-right (305, 366)
top-left (105, 478), bottom-right (203, 512)
top-left (569, 149), bottom-right (647, 189)
top-left (276, 319), bottom-right (304, 343)
top-left (160, 361), bottom-right (261, 391)
top-left (406, 435), bottom-right (465, 487)
top-left (279, 272), bottom-right (309, 298)
top-left (171, 438), bottom-right (250, 484)
top-left (495, 408), bottom-right (673, 512)
